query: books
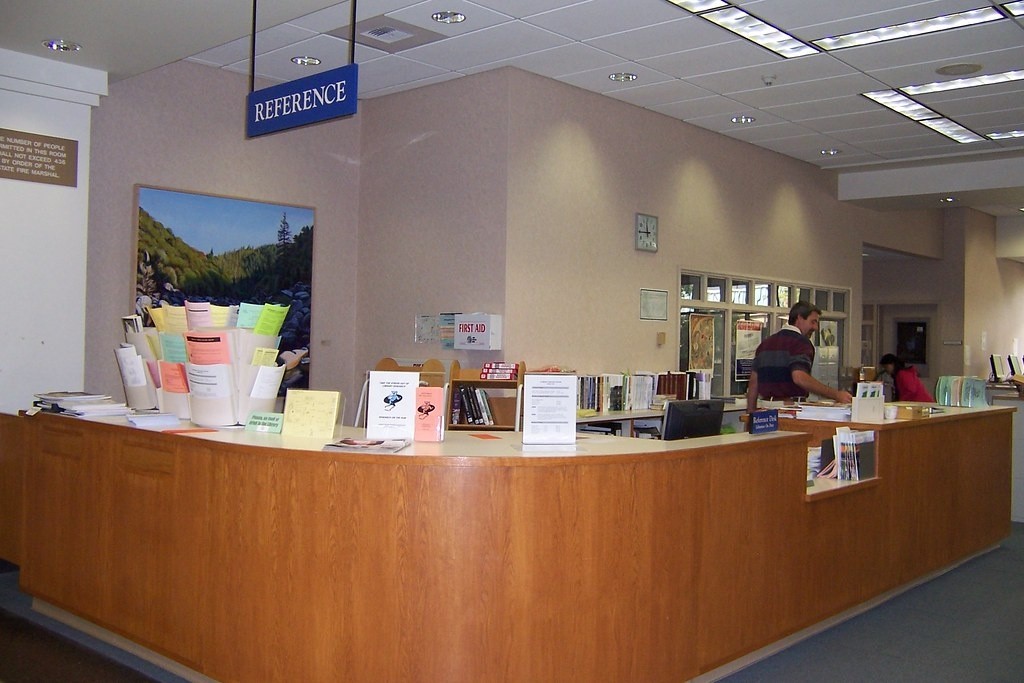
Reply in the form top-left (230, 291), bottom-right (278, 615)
top-left (33, 391), bottom-right (180, 427)
top-left (577, 370), bottom-right (712, 440)
top-left (366, 370), bottom-right (444, 441)
top-left (451, 361), bottom-right (519, 426)
top-left (757, 402), bottom-right (875, 481)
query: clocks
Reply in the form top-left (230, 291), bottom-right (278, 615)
top-left (635, 212), bottom-right (659, 253)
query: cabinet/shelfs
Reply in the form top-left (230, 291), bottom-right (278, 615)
top-left (375, 358), bottom-right (526, 431)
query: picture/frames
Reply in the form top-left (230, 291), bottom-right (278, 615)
top-left (129, 183), bottom-right (316, 400)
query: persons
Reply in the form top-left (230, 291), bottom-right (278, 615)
top-left (746, 300), bottom-right (853, 414)
top-left (879, 353), bottom-right (936, 403)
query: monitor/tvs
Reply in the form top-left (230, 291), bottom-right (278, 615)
top-left (661, 399), bottom-right (724, 440)
top-left (1008, 355), bottom-right (1022, 376)
top-left (988, 355), bottom-right (1004, 383)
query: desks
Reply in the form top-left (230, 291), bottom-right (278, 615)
top-left (985, 384), bottom-right (1024, 523)
top-left (0, 402), bottom-right (1018, 683)
